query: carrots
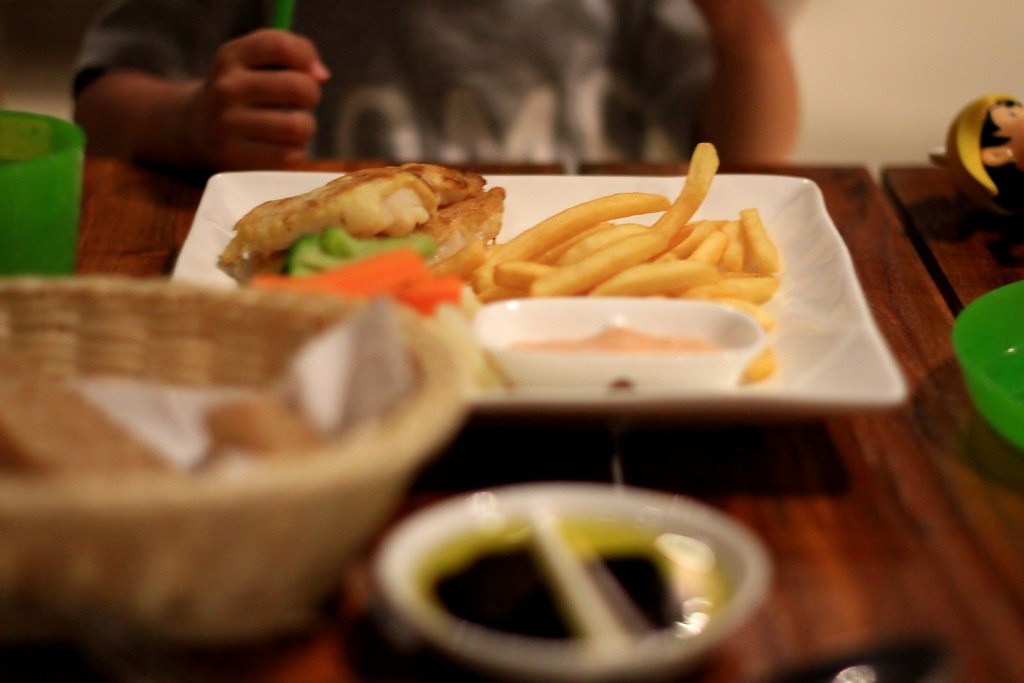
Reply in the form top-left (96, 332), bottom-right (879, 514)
top-left (250, 247), bottom-right (462, 315)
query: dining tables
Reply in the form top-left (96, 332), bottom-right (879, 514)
top-left (69, 144), bottom-right (1024, 683)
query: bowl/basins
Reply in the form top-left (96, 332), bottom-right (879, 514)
top-left (2, 280), bottom-right (471, 661)
top-left (469, 296), bottom-right (767, 389)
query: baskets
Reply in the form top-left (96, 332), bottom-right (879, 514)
top-left (0, 271), bottom-right (488, 649)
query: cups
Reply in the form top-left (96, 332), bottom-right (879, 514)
top-left (0, 108), bottom-right (87, 278)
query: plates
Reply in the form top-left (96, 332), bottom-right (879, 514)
top-left (167, 172), bottom-right (912, 429)
top-left (952, 281), bottom-right (1024, 448)
top-left (374, 480), bottom-right (775, 683)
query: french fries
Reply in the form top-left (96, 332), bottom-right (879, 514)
top-left (426, 140), bottom-right (782, 387)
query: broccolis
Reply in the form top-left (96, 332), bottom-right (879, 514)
top-left (280, 226), bottom-right (436, 275)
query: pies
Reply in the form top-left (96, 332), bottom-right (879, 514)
top-left (218, 163), bottom-right (509, 286)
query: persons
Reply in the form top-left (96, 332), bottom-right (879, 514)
top-left (71, 0), bottom-right (804, 175)
top-left (927, 94), bottom-right (1024, 209)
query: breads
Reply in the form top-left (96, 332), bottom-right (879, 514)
top-left (0, 352), bottom-right (337, 484)
